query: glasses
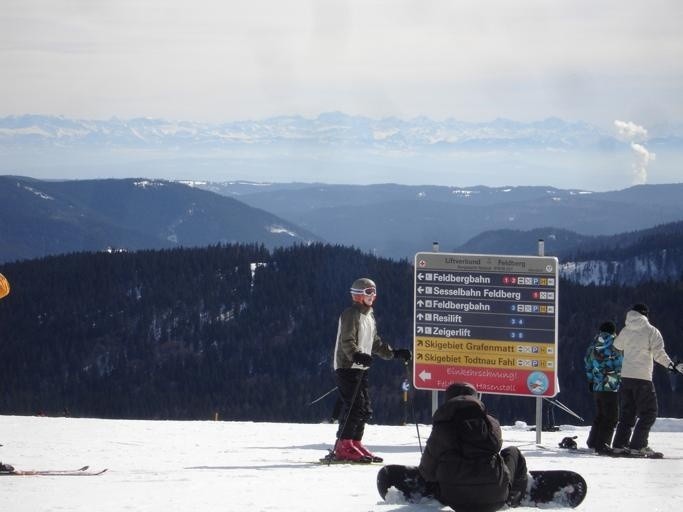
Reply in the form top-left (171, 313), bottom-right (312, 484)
top-left (362, 287), bottom-right (376, 297)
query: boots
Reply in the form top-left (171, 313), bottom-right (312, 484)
top-left (334, 438), bottom-right (372, 464)
top-left (352, 438), bottom-right (382, 463)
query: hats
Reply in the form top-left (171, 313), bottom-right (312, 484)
top-left (598, 319), bottom-right (617, 336)
top-left (631, 303), bottom-right (651, 319)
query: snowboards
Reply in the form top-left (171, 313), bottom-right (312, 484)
top-left (376, 465), bottom-right (587, 509)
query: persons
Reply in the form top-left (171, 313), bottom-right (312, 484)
top-left (332, 276), bottom-right (411, 461)
top-left (418, 381), bottom-right (528, 512)
top-left (0, 273), bottom-right (14, 471)
top-left (329, 396), bottom-right (342, 424)
top-left (583, 320), bottom-right (623, 455)
top-left (613, 302), bottom-right (683, 459)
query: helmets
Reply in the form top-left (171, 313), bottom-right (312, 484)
top-left (349, 277), bottom-right (376, 295)
top-left (445, 381), bottom-right (480, 405)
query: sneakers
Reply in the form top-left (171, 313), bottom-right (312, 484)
top-left (639, 445), bottom-right (664, 458)
top-left (609, 445), bottom-right (641, 457)
top-left (597, 442), bottom-right (613, 456)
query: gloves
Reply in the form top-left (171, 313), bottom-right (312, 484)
top-left (352, 350), bottom-right (374, 369)
top-left (393, 346), bottom-right (413, 364)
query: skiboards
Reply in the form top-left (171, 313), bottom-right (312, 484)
top-left (253, 456), bottom-right (389, 466)
top-left (535, 444), bottom-right (683, 460)
top-left (0, 465), bottom-right (108, 476)
